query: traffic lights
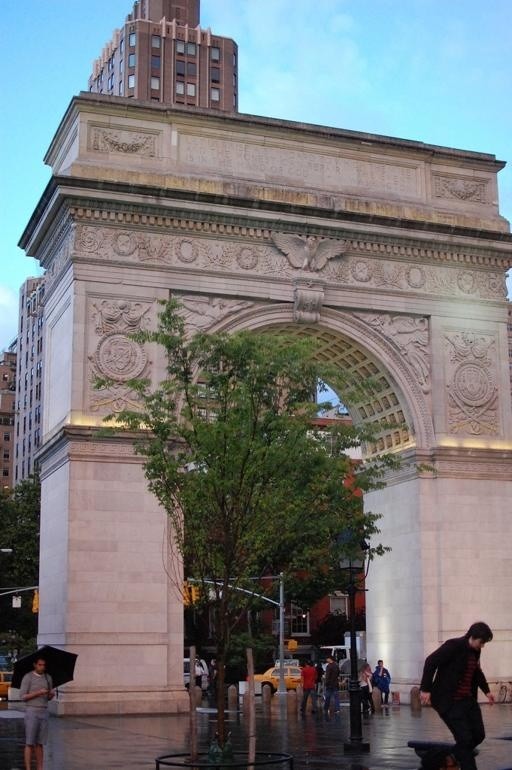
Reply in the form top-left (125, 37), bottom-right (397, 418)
top-left (190, 584), bottom-right (200, 605)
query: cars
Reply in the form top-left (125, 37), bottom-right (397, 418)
top-left (247, 658), bottom-right (309, 697)
top-left (0, 671), bottom-right (14, 699)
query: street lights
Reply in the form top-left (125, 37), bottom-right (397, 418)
top-left (333, 521), bottom-right (374, 755)
top-left (0, 548), bottom-right (15, 554)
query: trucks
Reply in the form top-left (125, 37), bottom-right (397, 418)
top-left (315, 629), bottom-right (366, 677)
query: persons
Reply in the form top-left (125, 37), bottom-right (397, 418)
top-left (17, 657), bottom-right (57, 770)
top-left (298, 654), bottom-right (393, 714)
top-left (208, 658), bottom-right (219, 700)
top-left (419, 622), bottom-right (496, 769)
top-left (194, 659), bottom-right (205, 687)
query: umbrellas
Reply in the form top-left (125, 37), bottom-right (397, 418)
top-left (9, 644), bottom-right (79, 690)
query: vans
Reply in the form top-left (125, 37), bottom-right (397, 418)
top-left (0, 656), bottom-right (13, 672)
top-left (182, 657), bottom-right (210, 695)
